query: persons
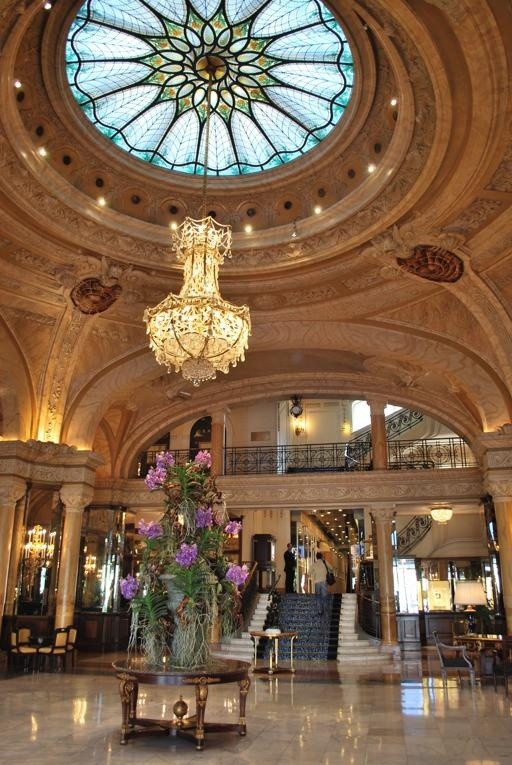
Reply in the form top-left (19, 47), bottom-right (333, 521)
top-left (283, 542), bottom-right (297, 594)
top-left (306, 552), bottom-right (333, 615)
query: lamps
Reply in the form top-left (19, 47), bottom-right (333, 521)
top-left (142, 58), bottom-right (254, 387)
top-left (428, 507), bottom-right (454, 526)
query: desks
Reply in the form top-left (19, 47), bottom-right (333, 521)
top-left (250, 631), bottom-right (298, 675)
top-left (112, 659), bottom-right (251, 752)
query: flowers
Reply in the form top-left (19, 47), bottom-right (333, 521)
top-left (118, 448), bottom-right (251, 669)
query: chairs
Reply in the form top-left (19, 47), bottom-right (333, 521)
top-left (434, 620), bottom-right (512, 696)
top-left (11, 625), bottom-right (80, 673)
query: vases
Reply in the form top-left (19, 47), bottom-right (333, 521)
top-left (159, 574), bottom-right (209, 669)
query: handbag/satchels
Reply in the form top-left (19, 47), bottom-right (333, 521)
top-left (326, 572), bottom-right (335, 584)
